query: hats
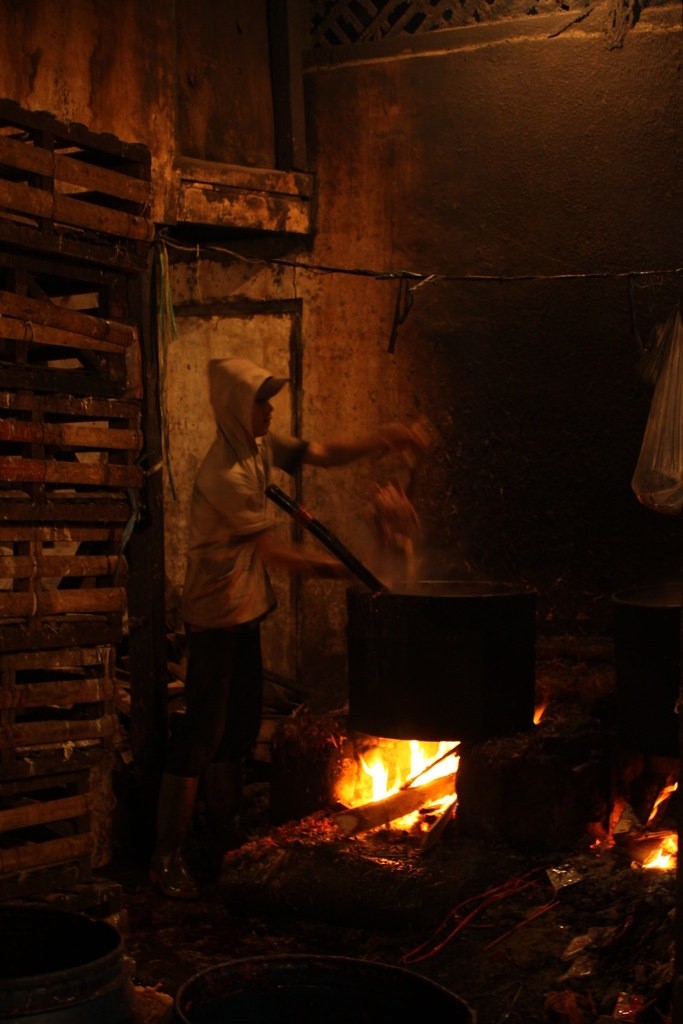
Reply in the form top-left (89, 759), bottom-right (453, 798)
top-left (255, 377), bottom-right (291, 402)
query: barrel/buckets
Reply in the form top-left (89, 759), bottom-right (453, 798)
top-left (0, 903), bottom-right (132, 1024)
top-left (171, 953), bottom-right (480, 1024)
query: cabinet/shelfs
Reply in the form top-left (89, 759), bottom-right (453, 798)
top-left (0, 98), bottom-right (153, 891)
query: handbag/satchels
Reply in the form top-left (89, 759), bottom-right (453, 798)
top-left (632, 302), bottom-right (683, 517)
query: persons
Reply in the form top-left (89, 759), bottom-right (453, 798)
top-left (184, 356), bottom-right (398, 882)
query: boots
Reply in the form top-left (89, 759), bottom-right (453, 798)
top-left (146, 772), bottom-right (201, 899)
top-left (207, 760), bottom-right (244, 872)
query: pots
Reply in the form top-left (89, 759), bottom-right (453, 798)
top-left (344, 577), bottom-right (543, 739)
top-left (610, 584), bottom-right (682, 757)
